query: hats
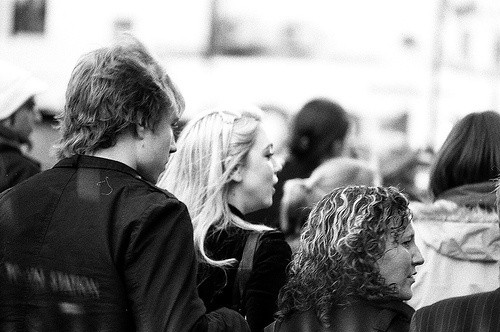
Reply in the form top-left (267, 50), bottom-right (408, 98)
top-left (0, 74), bottom-right (33, 121)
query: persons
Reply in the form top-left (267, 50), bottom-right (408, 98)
top-left (260, 98), bottom-right (434, 247)
top-left (0, 61), bottom-right (44, 191)
top-left (262, 185), bottom-right (425, 332)
top-left (0, 40), bottom-right (252, 332)
top-left (403, 110), bottom-right (500, 310)
top-left (156, 110), bottom-right (287, 331)
top-left (411, 290), bottom-right (500, 332)
